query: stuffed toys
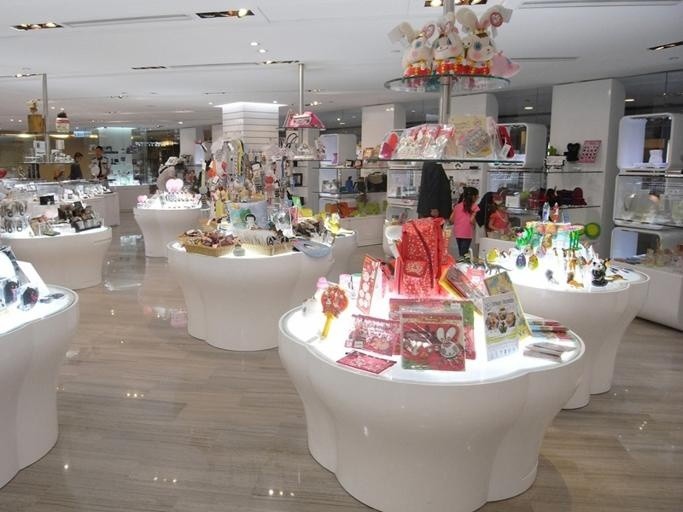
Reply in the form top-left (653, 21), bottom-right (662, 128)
top-left (432, 11), bottom-right (465, 83)
top-left (399, 21), bottom-right (435, 89)
top-left (456, 5), bottom-right (513, 90)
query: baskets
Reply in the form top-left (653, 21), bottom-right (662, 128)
top-left (178, 234), bottom-right (236, 257)
top-left (241, 241), bottom-right (290, 256)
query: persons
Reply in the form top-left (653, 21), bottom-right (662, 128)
top-left (448, 187), bottom-right (480, 257)
top-left (415, 159), bottom-right (452, 233)
top-left (89, 146), bottom-right (110, 189)
top-left (69, 151), bottom-right (83, 179)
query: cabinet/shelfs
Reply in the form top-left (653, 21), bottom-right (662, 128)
top-left (280, 113), bottom-right (681, 278)
